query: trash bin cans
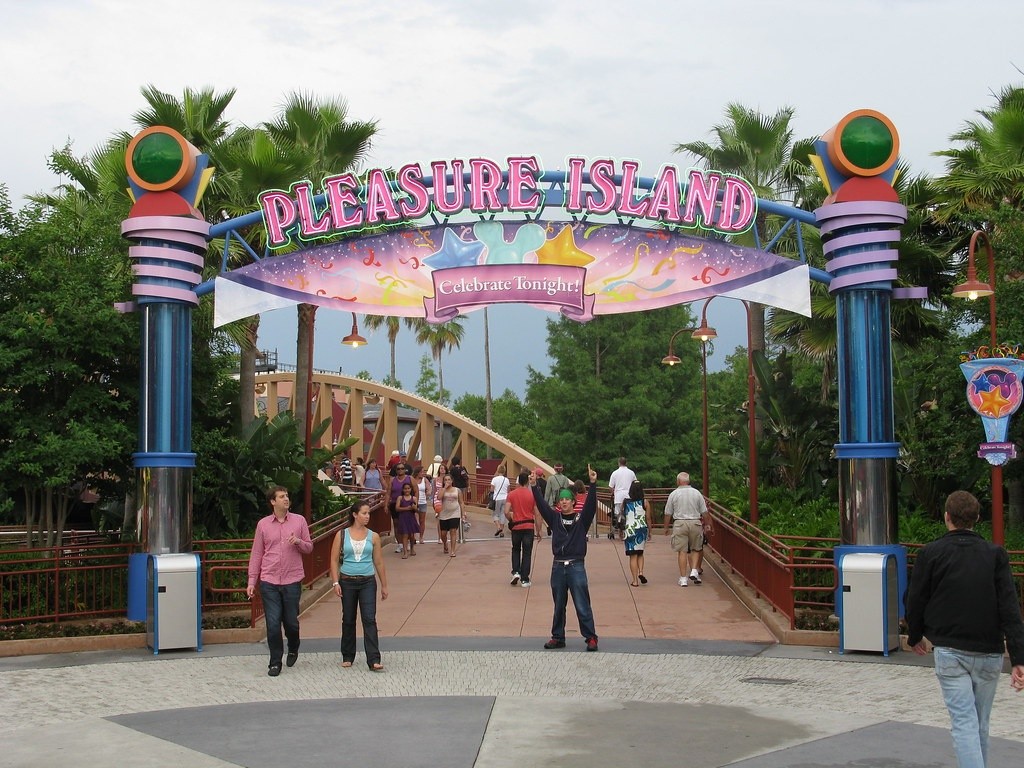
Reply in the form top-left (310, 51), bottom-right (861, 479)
top-left (838, 554), bottom-right (899, 657)
top-left (145, 553), bottom-right (202, 655)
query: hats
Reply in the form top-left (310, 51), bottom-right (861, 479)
top-left (531, 469), bottom-right (542, 476)
top-left (399, 451), bottom-right (406, 456)
top-left (555, 464), bottom-right (562, 472)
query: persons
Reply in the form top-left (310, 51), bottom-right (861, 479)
top-left (322, 449), bottom-right (472, 556)
top-left (530, 464), bottom-right (598, 650)
top-left (247, 486), bottom-right (314, 677)
top-left (619, 480), bottom-right (652, 587)
top-left (906, 491), bottom-right (1024, 768)
top-left (515, 463), bottom-right (589, 539)
top-left (609, 457), bottom-right (637, 541)
top-left (664, 472), bottom-right (715, 586)
top-left (503, 472), bottom-right (542, 588)
top-left (489, 464), bottom-right (510, 537)
top-left (331, 499), bottom-right (389, 671)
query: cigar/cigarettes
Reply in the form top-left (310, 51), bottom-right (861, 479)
top-left (248, 597), bottom-right (251, 600)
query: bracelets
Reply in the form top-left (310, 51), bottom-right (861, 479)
top-left (332, 582), bottom-right (339, 586)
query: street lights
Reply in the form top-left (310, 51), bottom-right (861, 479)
top-left (952, 230), bottom-right (1006, 546)
top-left (691, 295), bottom-right (760, 527)
top-left (305, 305), bottom-right (369, 530)
top-left (662, 327), bottom-right (709, 499)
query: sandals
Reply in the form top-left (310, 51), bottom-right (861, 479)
top-left (343, 662), bottom-right (351, 667)
top-left (372, 663), bottom-right (383, 671)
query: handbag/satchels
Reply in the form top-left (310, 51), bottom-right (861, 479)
top-left (508, 520), bottom-right (535, 530)
top-left (617, 498), bottom-right (627, 529)
top-left (337, 529), bottom-right (344, 564)
top-left (488, 501), bottom-right (496, 511)
top-left (458, 467), bottom-right (468, 484)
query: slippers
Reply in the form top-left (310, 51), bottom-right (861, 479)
top-left (631, 580), bottom-right (637, 587)
top-left (638, 574), bottom-right (647, 584)
top-left (451, 550), bottom-right (456, 557)
top-left (444, 545), bottom-right (448, 553)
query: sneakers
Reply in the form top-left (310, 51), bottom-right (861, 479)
top-left (287, 651), bottom-right (296, 666)
top-left (677, 577), bottom-right (688, 587)
top-left (586, 638), bottom-right (598, 651)
top-left (690, 569), bottom-right (701, 584)
top-left (268, 665), bottom-right (280, 677)
top-left (544, 640), bottom-right (565, 650)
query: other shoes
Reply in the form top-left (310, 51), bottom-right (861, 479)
top-left (521, 581), bottom-right (531, 588)
top-left (395, 539), bottom-right (424, 559)
top-left (495, 528), bottom-right (504, 537)
top-left (547, 527), bottom-right (552, 536)
top-left (510, 574), bottom-right (520, 584)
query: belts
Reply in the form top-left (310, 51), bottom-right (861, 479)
top-left (341, 574), bottom-right (369, 579)
